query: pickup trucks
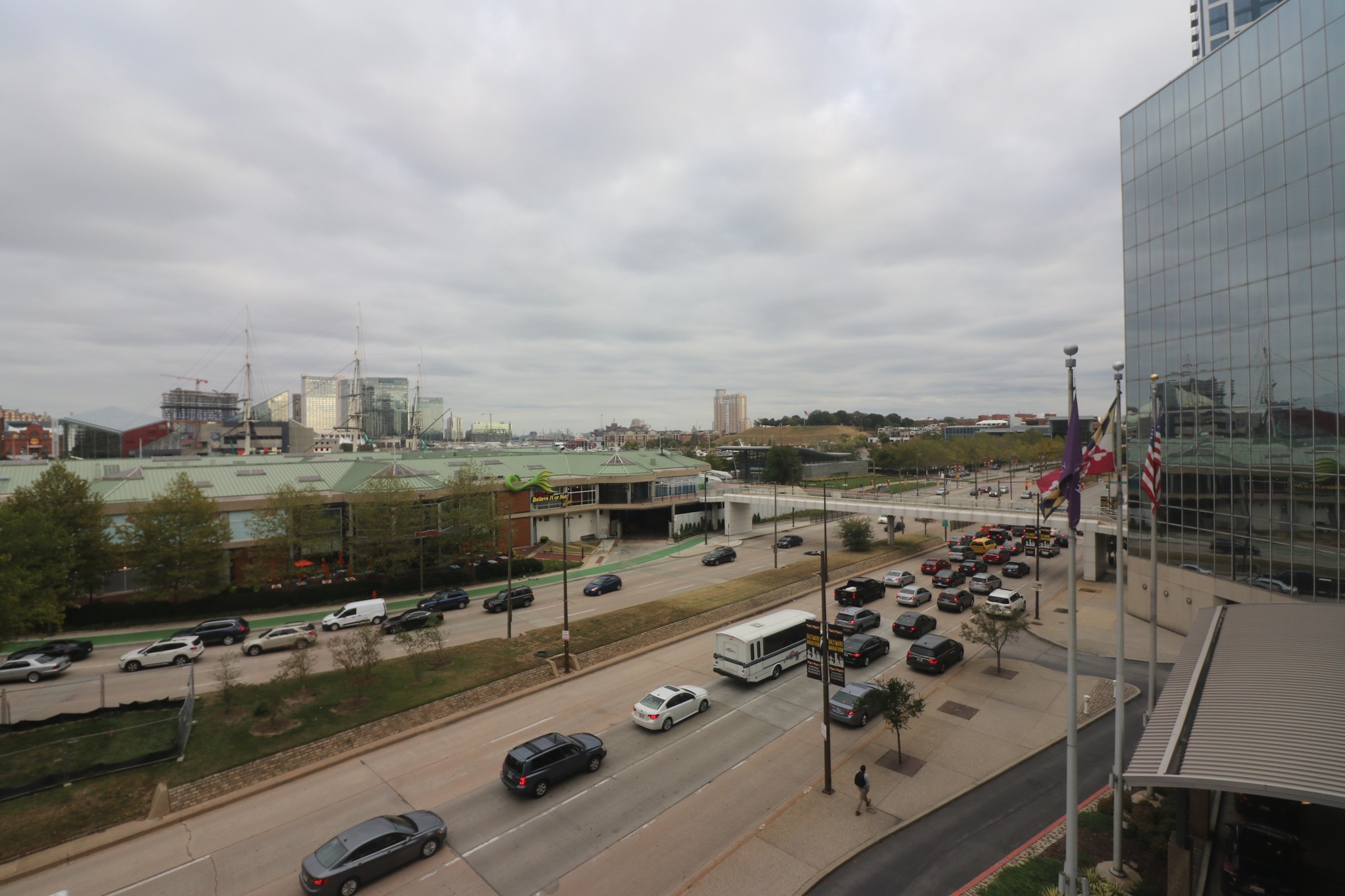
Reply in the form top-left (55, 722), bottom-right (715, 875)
top-left (833, 575), bottom-right (886, 607)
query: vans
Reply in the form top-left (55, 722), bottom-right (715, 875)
top-left (322, 597), bottom-right (388, 631)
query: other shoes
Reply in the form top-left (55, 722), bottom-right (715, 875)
top-left (866, 799), bottom-right (871, 806)
top-left (856, 811), bottom-right (861, 816)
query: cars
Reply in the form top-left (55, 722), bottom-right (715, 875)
top-left (299, 810), bottom-right (447, 896)
top-left (0, 641), bottom-right (95, 683)
top-left (584, 574), bottom-right (622, 596)
top-left (776, 534), bottom-right (803, 548)
top-left (380, 608), bottom-right (444, 634)
top-left (826, 463), bottom-right (1069, 727)
top-left (630, 684), bottom-right (711, 731)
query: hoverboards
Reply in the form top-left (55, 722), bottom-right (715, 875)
top-left (519, 567), bottom-right (538, 580)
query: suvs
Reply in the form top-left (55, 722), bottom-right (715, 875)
top-left (500, 732), bottom-right (608, 798)
top-left (118, 618), bottom-right (317, 672)
top-left (702, 547), bottom-right (736, 566)
top-left (417, 585), bottom-right (534, 613)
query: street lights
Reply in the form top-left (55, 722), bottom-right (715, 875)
top-left (701, 472), bottom-right (707, 545)
top-left (418, 494), bottom-right (425, 595)
top-left (792, 467), bottom-right (797, 527)
top-left (804, 550), bottom-right (835, 795)
top-left (909, 454), bottom-right (921, 497)
top-left (503, 506), bottom-right (511, 639)
top-left (563, 515), bottom-right (574, 674)
top-left (957, 448), bottom-right (989, 489)
top-left (771, 481), bottom-right (777, 568)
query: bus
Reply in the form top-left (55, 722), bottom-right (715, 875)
top-left (712, 609), bottom-right (823, 683)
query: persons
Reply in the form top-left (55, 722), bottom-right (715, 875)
top-left (875, 492), bottom-right (879, 497)
top-left (888, 479), bottom-right (890, 485)
top-left (942, 492), bottom-right (944, 498)
top-left (795, 481), bottom-right (807, 489)
top-left (854, 765), bottom-right (871, 815)
top-left (916, 490), bottom-right (920, 496)
top-left (890, 491), bottom-right (892, 497)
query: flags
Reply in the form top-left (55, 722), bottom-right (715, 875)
top-left (1141, 397), bottom-right (1161, 515)
top-left (1059, 393), bottom-right (1084, 530)
top-left (1036, 396), bottom-right (1116, 521)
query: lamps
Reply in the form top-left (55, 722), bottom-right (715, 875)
top-left (1186, 598), bottom-right (1191, 604)
top-left (1163, 591), bottom-right (1168, 596)
top-left (1142, 584), bottom-right (1147, 590)
top-left (579, 515), bottom-right (582, 518)
top-left (538, 518), bottom-right (543, 521)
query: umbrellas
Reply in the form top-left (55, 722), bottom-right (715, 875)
top-left (293, 559), bottom-right (314, 567)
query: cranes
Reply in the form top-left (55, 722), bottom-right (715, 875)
top-left (160, 375), bottom-right (209, 423)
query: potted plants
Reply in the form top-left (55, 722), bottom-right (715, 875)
top-left (540, 536), bottom-right (549, 544)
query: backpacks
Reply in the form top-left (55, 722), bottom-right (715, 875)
top-left (854, 771), bottom-right (865, 787)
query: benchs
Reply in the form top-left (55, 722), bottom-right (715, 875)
top-left (581, 534), bottom-right (595, 540)
